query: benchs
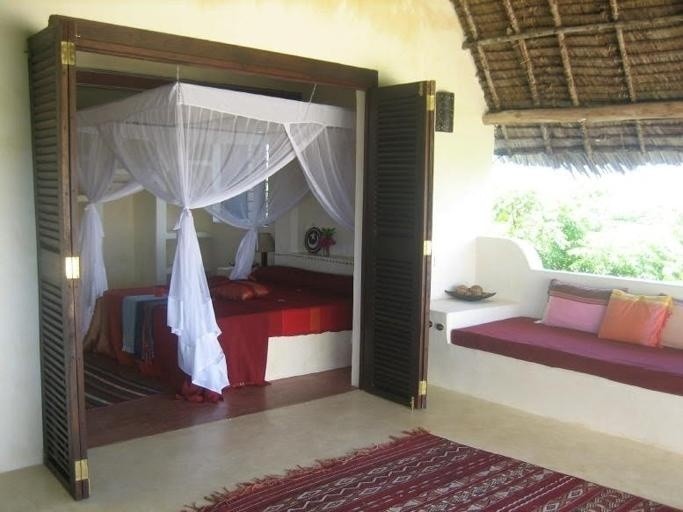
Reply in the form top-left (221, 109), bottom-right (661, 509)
top-left (428, 235), bottom-right (682, 455)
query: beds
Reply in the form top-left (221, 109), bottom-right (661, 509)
top-left (102, 264), bottom-right (351, 392)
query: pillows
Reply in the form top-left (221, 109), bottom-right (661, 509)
top-left (532, 279), bottom-right (682, 351)
top-left (206, 276), bottom-right (269, 303)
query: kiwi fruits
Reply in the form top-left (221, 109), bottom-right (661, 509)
top-left (456, 285), bottom-right (484, 296)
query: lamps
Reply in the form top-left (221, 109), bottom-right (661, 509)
top-left (255, 233), bottom-right (274, 266)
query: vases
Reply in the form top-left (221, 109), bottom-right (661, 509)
top-left (323, 246), bottom-right (329, 257)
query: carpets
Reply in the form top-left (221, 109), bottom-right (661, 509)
top-left (175, 427), bottom-right (682, 512)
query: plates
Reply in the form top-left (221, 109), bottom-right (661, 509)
top-left (443, 290), bottom-right (496, 301)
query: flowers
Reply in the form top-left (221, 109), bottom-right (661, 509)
top-left (320, 226), bottom-right (336, 257)
